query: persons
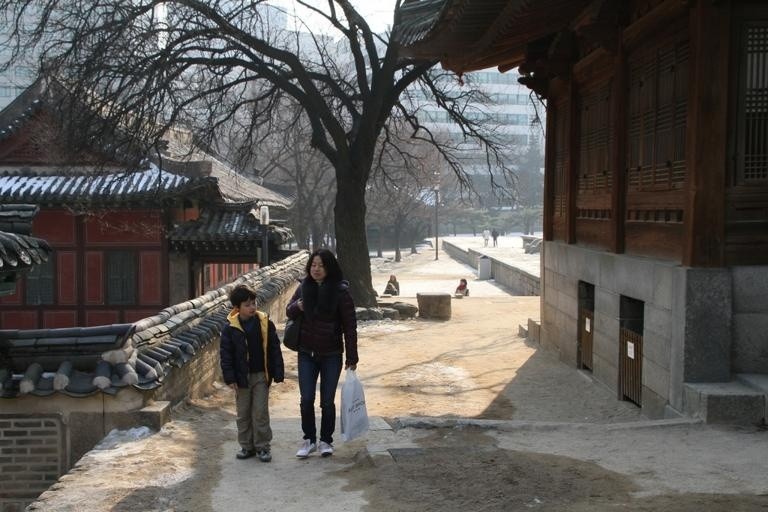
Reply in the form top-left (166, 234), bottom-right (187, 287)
top-left (491, 227), bottom-right (499, 248)
top-left (481, 227), bottom-right (490, 248)
top-left (285, 247), bottom-right (359, 458)
top-left (219, 283), bottom-right (287, 462)
top-left (384, 274), bottom-right (399, 296)
top-left (454, 278), bottom-right (470, 296)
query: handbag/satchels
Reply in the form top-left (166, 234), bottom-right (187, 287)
top-left (283, 320), bottom-right (301, 351)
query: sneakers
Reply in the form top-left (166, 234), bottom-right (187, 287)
top-left (236, 448), bottom-right (272, 462)
top-left (296, 439), bottom-right (334, 457)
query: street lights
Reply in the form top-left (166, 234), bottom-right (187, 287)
top-left (433, 172), bottom-right (440, 260)
